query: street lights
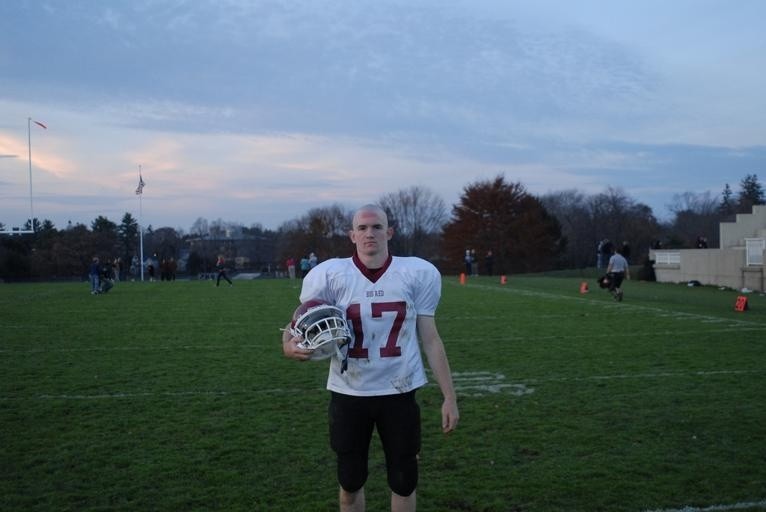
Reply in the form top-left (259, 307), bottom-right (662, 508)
top-left (26, 117), bottom-right (47, 231)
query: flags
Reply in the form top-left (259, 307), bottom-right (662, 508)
top-left (134, 171), bottom-right (144, 194)
top-left (29, 118), bottom-right (46, 129)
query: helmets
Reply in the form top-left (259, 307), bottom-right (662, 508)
top-left (290, 298), bottom-right (353, 361)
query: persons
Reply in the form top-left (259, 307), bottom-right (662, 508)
top-left (286, 252), bottom-right (317, 279)
top-left (464, 248), bottom-right (493, 277)
top-left (102, 255), bottom-right (176, 282)
top-left (602, 248), bottom-right (632, 301)
top-left (654, 239), bottom-right (664, 250)
top-left (696, 236), bottom-right (708, 249)
top-left (89, 257), bottom-right (112, 295)
top-left (282, 203), bottom-right (458, 510)
top-left (214, 255), bottom-right (231, 287)
